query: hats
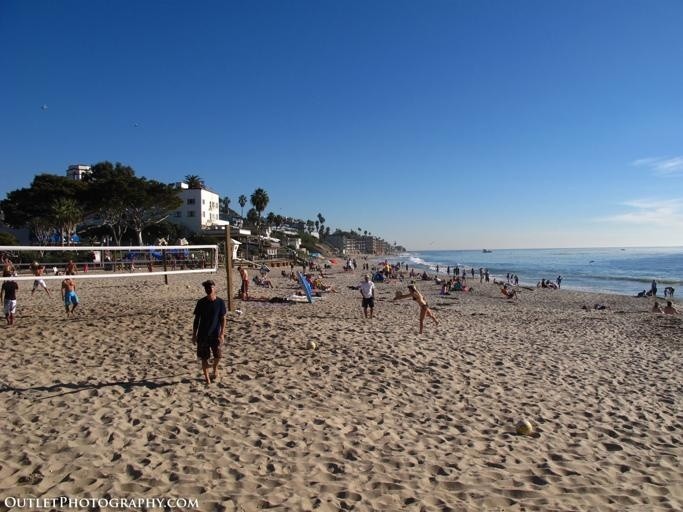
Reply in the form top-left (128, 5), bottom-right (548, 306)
top-left (202, 281), bottom-right (215, 285)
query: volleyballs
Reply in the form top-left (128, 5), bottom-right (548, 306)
top-left (395, 290), bottom-right (402, 298)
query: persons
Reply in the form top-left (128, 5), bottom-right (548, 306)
top-left (391, 284), bottom-right (439, 334)
top-left (29, 263), bottom-right (55, 300)
top-left (635, 278), bottom-right (678, 315)
top-left (60, 270), bottom-right (80, 318)
top-left (191, 280), bottom-right (227, 386)
top-left (359, 274), bottom-right (376, 319)
top-left (0, 273), bottom-right (18, 325)
top-left (0, 250), bottom-right (208, 290)
top-left (236, 252), bottom-right (563, 301)
